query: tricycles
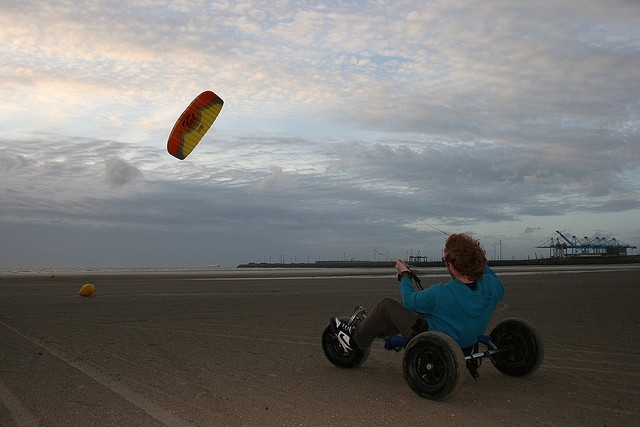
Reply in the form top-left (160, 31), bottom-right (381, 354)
top-left (322, 305), bottom-right (546, 402)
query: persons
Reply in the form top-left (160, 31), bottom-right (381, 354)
top-left (330, 232), bottom-right (505, 356)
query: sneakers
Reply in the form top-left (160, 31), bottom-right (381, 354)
top-left (330, 317), bottom-right (360, 356)
